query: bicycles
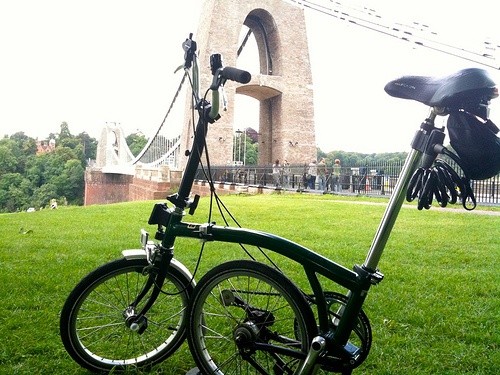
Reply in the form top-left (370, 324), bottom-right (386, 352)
top-left (59, 32), bottom-right (500, 375)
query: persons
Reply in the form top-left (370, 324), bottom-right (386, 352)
top-left (307, 160), bottom-right (317, 190)
top-left (282, 160), bottom-right (291, 189)
top-left (331, 159), bottom-right (342, 193)
top-left (273, 159), bottom-right (281, 188)
top-left (50, 199), bottom-right (57, 209)
top-left (317, 158), bottom-right (328, 191)
top-left (222, 169), bottom-right (248, 184)
